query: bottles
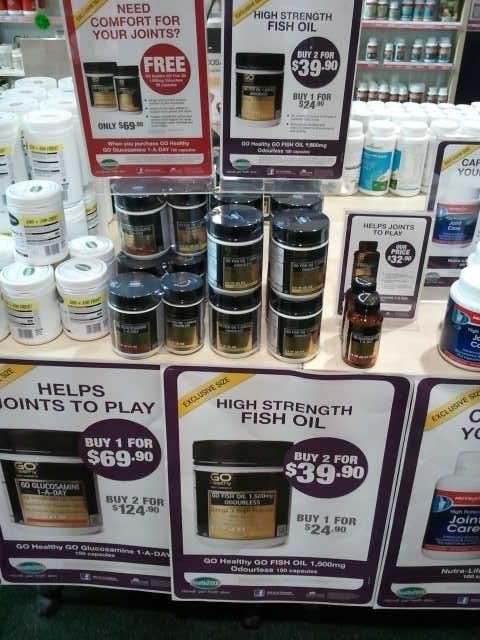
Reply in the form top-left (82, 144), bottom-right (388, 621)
top-left (328, 100), bottom-right (480, 197)
top-left (352, 1), bottom-right (463, 103)
top-left (420, 448), bottom-right (480, 561)
top-left (0, 427), bottom-right (107, 536)
top-left (187, 437), bottom-right (294, 553)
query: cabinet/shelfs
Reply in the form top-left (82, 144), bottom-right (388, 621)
top-left (353, 0), bottom-right (473, 102)
